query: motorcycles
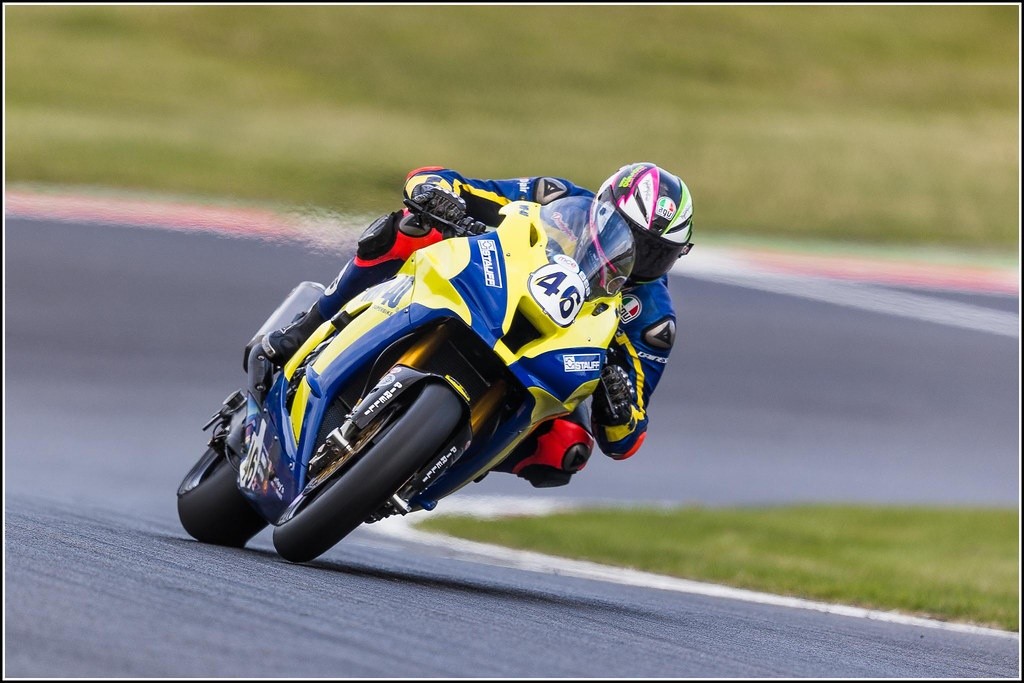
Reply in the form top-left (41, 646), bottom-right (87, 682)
top-left (176, 195), bottom-right (619, 565)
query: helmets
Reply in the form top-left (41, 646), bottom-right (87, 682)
top-left (590, 162), bottom-right (693, 294)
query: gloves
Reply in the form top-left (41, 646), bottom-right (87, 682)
top-left (414, 186), bottom-right (465, 226)
top-left (594, 364), bottom-right (635, 426)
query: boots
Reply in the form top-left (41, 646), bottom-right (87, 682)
top-left (261, 301), bottom-right (326, 362)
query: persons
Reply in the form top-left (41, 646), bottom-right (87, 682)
top-left (262, 163), bottom-right (695, 488)
